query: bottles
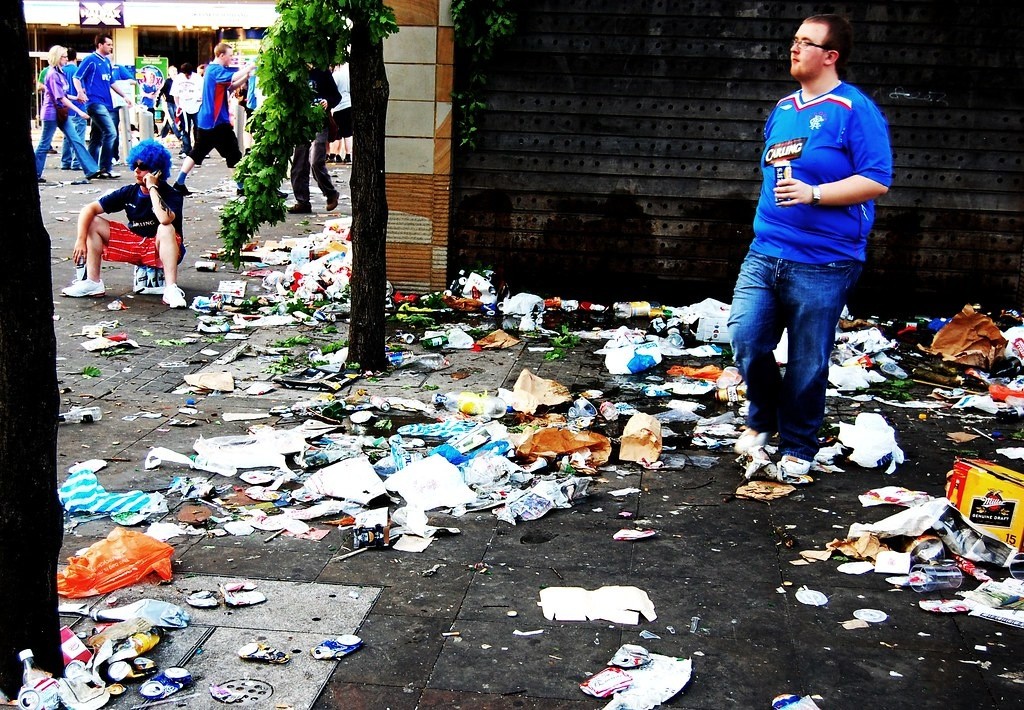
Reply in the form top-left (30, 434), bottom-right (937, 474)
top-left (19, 649), bottom-right (60, 710)
top-left (58, 407), bottom-right (102, 423)
top-left (933, 388), bottom-right (964, 396)
top-left (389, 352), bottom-right (413, 361)
top-left (450, 266), bottom-right (466, 297)
top-left (322, 400), bottom-right (346, 417)
top-left (422, 336), bottom-right (449, 350)
top-left (614, 302), bottom-right (666, 318)
top-left (890, 318), bottom-right (925, 329)
top-left (90, 599), bottom-right (190, 627)
top-left (194, 260), bottom-right (218, 271)
top-left (317, 252), bottom-right (346, 277)
top-left (304, 450), bottom-right (348, 466)
top-left (874, 351), bottom-right (907, 379)
top-left (715, 385), bottom-right (748, 402)
top-left (370, 394), bottom-right (391, 411)
top-left (309, 351), bottom-right (318, 362)
top-left (431, 392), bottom-right (506, 418)
top-left (599, 401), bottom-right (619, 420)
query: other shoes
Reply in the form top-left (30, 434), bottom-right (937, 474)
top-left (336, 155), bottom-right (342, 162)
top-left (194, 165), bottom-right (201, 168)
top-left (178, 153), bottom-right (187, 158)
top-left (48, 149), bottom-right (57, 154)
top-left (38, 178), bottom-right (46, 183)
top-left (173, 181), bottom-right (192, 195)
top-left (345, 154), bottom-right (351, 161)
top-left (205, 155), bottom-right (210, 158)
top-left (102, 170), bottom-right (121, 178)
top-left (237, 188), bottom-right (244, 196)
top-left (325, 154), bottom-right (329, 161)
top-left (329, 154), bottom-right (336, 160)
top-left (86, 169), bottom-right (109, 180)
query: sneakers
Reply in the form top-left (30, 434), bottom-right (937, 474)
top-left (62, 279), bottom-right (105, 298)
top-left (287, 204), bottom-right (312, 213)
top-left (781, 455), bottom-right (810, 475)
top-left (735, 428), bottom-right (768, 454)
top-left (326, 192), bottom-right (340, 211)
top-left (163, 283), bottom-right (187, 308)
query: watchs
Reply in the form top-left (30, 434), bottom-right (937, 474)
top-left (810, 186), bottom-right (820, 204)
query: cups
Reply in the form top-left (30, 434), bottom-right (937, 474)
top-left (909, 564), bottom-right (962, 592)
top-left (1009, 550), bottom-right (1024, 579)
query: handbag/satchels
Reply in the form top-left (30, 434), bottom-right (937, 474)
top-left (56, 106), bottom-right (69, 123)
top-left (327, 110), bottom-right (338, 142)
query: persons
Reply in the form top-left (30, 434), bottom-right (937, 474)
top-left (35, 33), bottom-right (132, 182)
top-left (62, 137), bottom-right (187, 309)
top-left (173, 43), bottom-right (257, 195)
top-left (137, 53), bottom-right (352, 164)
top-left (287, 62), bottom-right (342, 214)
top-left (727, 14), bottom-right (892, 475)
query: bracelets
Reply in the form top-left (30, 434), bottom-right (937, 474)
top-left (149, 185), bottom-right (158, 191)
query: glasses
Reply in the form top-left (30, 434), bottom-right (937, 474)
top-left (130, 162), bottom-right (149, 171)
top-left (62, 56), bottom-right (67, 58)
top-left (793, 40), bottom-right (822, 49)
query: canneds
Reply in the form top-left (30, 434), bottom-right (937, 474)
top-left (648, 308), bottom-right (673, 319)
top-left (387, 351), bottom-right (412, 365)
top-left (773, 160), bottom-right (792, 203)
top-left (136, 266), bottom-right (165, 288)
top-left (17, 581), bottom-right (362, 710)
top-left (195, 261), bottom-right (217, 272)
top-left (370, 395), bottom-right (390, 412)
top-left (195, 298), bottom-right (222, 310)
